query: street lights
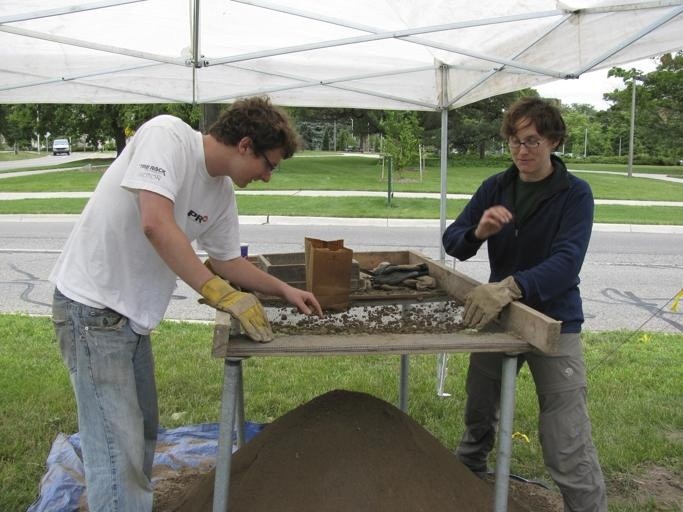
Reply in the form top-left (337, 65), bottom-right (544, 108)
top-left (627, 75), bottom-right (645, 178)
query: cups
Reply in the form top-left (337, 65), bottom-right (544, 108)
top-left (240, 243), bottom-right (249, 258)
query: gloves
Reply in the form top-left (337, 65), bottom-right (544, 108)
top-left (201, 275), bottom-right (276, 342)
top-left (372, 261), bottom-right (429, 287)
top-left (460, 273), bottom-right (523, 332)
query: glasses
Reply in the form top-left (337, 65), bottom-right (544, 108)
top-left (505, 136), bottom-right (546, 150)
top-left (254, 145), bottom-right (277, 172)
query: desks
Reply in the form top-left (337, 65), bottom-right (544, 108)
top-left (211, 250), bottom-right (563, 512)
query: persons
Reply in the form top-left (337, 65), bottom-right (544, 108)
top-left (51, 96), bottom-right (324, 512)
top-left (442, 99), bottom-right (608, 512)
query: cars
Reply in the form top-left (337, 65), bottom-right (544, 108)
top-left (552, 151), bottom-right (576, 159)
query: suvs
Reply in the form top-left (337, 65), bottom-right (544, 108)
top-left (52, 139), bottom-right (69, 155)
